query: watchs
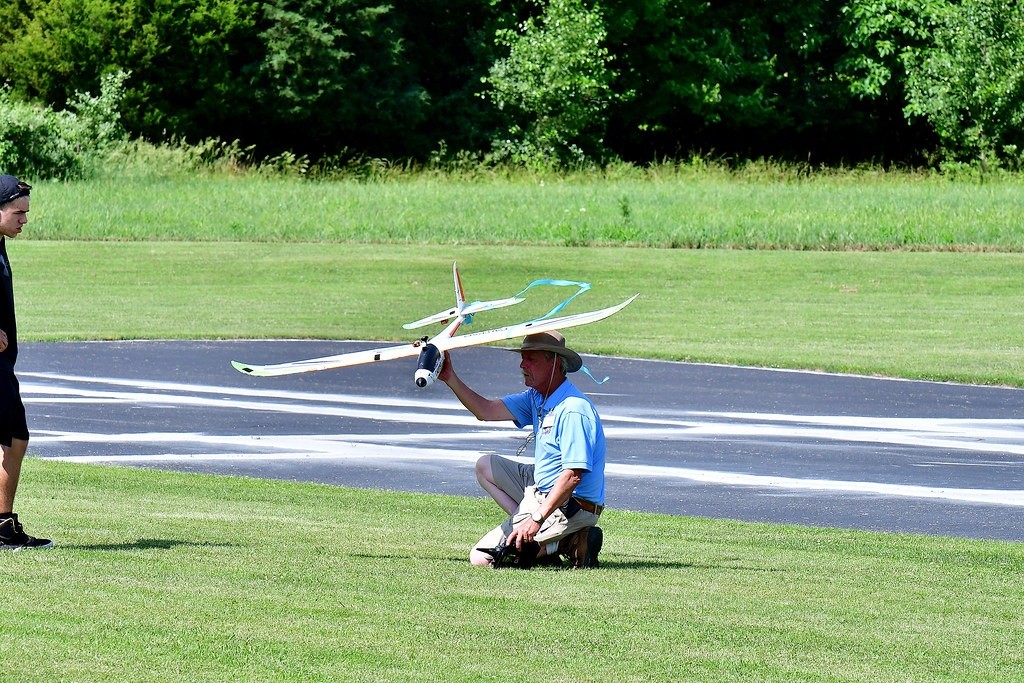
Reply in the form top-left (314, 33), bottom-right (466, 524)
top-left (531, 511), bottom-right (545, 525)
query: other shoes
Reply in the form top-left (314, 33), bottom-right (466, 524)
top-left (571, 525), bottom-right (604, 568)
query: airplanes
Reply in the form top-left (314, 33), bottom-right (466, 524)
top-left (230, 259), bottom-right (640, 389)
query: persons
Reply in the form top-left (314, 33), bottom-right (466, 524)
top-left (0, 174), bottom-right (54, 550)
top-left (438, 330), bottom-right (608, 569)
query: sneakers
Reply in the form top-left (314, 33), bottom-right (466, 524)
top-left (0, 513), bottom-right (54, 549)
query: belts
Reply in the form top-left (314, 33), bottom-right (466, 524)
top-left (578, 497), bottom-right (604, 516)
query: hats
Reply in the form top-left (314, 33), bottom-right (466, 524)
top-left (503, 329), bottom-right (583, 373)
top-left (0, 175), bottom-right (30, 205)
